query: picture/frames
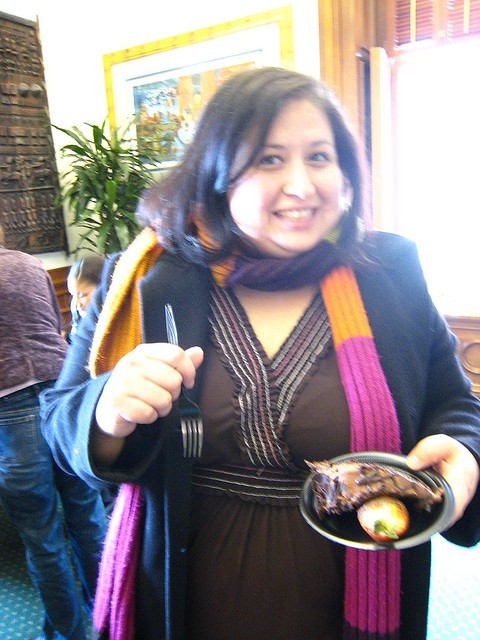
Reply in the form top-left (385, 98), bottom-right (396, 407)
top-left (101, 2), bottom-right (297, 190)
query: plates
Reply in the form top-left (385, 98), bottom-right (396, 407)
top-left (298, 450), bottom-right (455, 554)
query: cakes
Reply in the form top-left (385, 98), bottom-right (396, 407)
top-left (303, 458), bottom-right (446, 521)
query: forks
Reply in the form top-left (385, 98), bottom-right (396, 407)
top-left (164, 300), bottom-right (205, 460)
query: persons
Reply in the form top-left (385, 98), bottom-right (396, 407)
top-left (38, 66), bottom-right (479, 640)
top-left (0, 236), bottom-right (108, 640)
top-left (65, 257), bottom-right (101, 341)
